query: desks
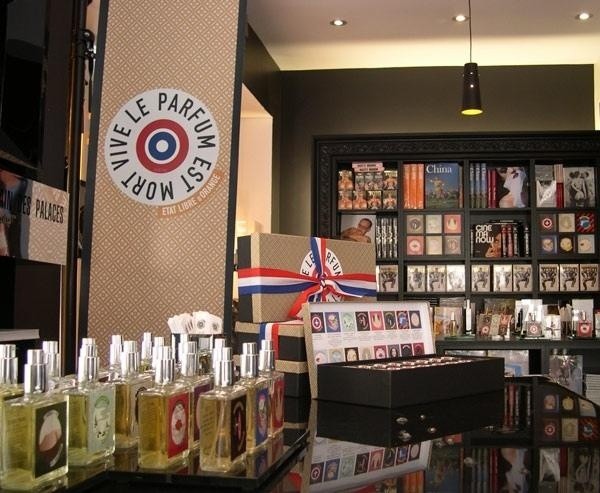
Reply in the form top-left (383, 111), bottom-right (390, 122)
top-left (0, 376), bottom-right (600, 492)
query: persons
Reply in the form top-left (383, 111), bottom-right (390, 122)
top-left (337, 170), bottom-right (398, 211)
top-left (564, 170), bottom-right (589, 209)
top-left (380, 266), bottom-right (597, 291)
top-left (341, 218), bottom-right (372, 242)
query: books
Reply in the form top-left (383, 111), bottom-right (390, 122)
top-left (468, 214), bottom-right (532, 258)
top-left (402, 162), bottom-right (465, 208)
top-left (554, 164), bottom-right (563, 209)
top-left (496, 384), bottom-right (531, 435)
top-left (463, 447), bottom-right (499, 492)
top-left (375, 217), bottom-right (397, 259)
top-left (467, 162), bottom-right (498, 208)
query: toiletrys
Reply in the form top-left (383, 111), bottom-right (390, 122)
top-left (577, 311), bottom-right (591, 337)
top-left (526, 313), bottom-right (541, 337)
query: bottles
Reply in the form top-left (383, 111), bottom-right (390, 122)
top-left (525, 310), bottom-right (543, 337)
top-left (0, 331), bottom-right (286, 493)
top-left (577, 311), bottom-right (592, 338)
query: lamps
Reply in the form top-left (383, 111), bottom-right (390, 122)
top-left (461, 1), bottom-right (482, 115)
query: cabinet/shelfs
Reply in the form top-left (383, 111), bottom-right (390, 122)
top-left (328, 151), bottom-right (600, 344)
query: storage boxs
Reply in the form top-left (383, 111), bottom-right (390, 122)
top-left (236, 232), bottom-right (375, 332)
top-left (318, 352), bottom-right (505, 450)
top-left (232, 321), bottom-right (313, 430)
